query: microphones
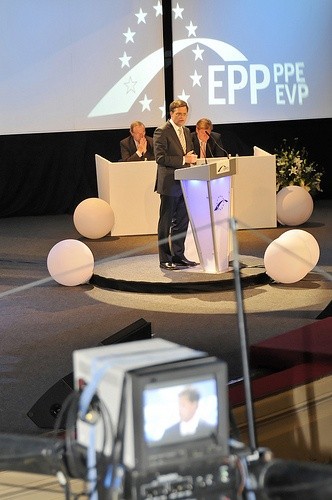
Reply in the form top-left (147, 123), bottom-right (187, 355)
top-left (193, 140), bottom-right (208, 164)
top-left (205, 130), bottom-right (229, 159)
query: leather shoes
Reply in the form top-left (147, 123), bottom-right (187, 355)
top-left (160, 257), bottom-right (196, 270)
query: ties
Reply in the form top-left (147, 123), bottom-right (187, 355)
top-left (178, 130), bottom-right (186, 152)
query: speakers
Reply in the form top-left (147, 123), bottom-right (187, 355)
top-left (29, 316), bottom-right (152, 430)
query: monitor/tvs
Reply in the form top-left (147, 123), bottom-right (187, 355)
top-left (73, 336), bottom-right (229, 475)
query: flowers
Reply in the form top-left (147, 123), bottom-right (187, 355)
top-left (270, 138), bottom-right (324, 192)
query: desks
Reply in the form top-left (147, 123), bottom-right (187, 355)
top-left (95, 146), bottom-right (278, 237)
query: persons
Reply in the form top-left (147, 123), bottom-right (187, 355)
top-left (191, 118), bottom-right (225, 159)
top-left (119, 121), bottom-right (156, 162)
top-left (161, 388), bottom-right (214, 441)
top-left (153, 100), bottom-right (196, 270)
